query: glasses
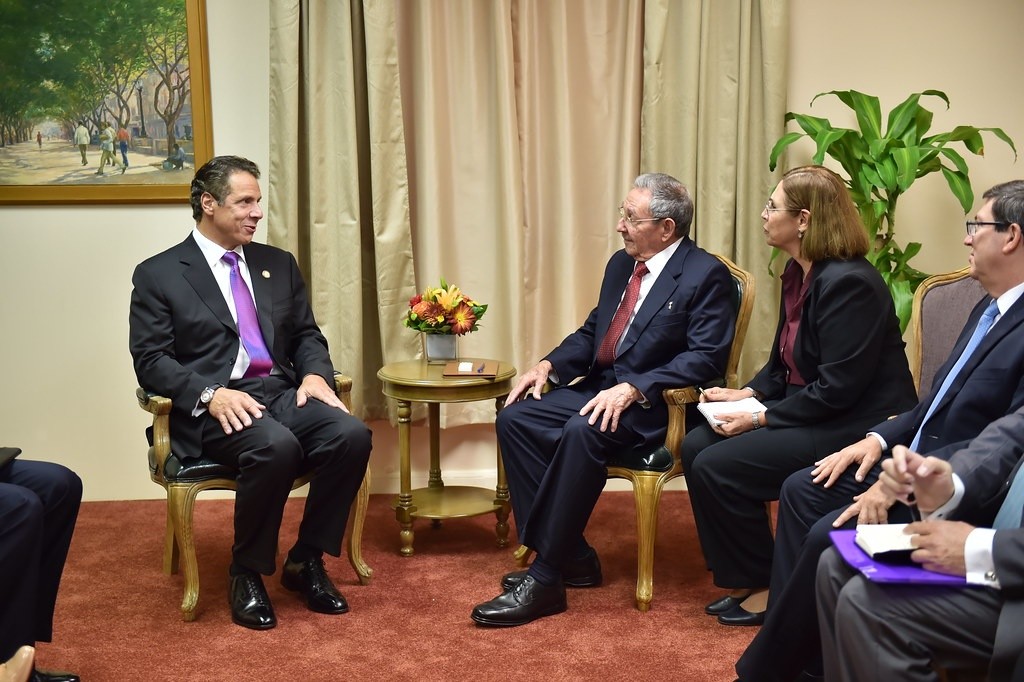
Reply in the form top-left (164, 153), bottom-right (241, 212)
top-left (766, 205), bottom-right (802, 216)
top-left (966, 220), bottom-right (1010, 236)
top-left (618, 207), bottom-right (678, 231)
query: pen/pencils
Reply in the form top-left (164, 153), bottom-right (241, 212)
top-left (478, 363), bottom-right (485, 373)
top-left (907, 482), bottom-right (922, 522)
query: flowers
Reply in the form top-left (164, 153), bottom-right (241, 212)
top-left (400, 275), bottom-right (488, 337)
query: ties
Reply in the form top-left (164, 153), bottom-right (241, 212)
top-left (220, 251), bottom-right (273, 380)
top-left (597, 261), bottom-right (650, 369)
top-left (992, 461), bottom-right (1024, 535)
top-left (909, 299), bottom-right (1001, 453)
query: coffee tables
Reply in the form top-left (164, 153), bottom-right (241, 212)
top-left (377, 359), bottom-right (516, 556)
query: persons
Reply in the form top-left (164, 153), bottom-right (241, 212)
top-left (725, 179), bottom-right (1024, 682)
top-left (0, 445), bottom-right (81, 682)
top-left (681, 165), bottom-right (920, 625)
top-left (469, 172), bottom-right (738, 627)
top-left (129, 156), bottom-right (372, 631)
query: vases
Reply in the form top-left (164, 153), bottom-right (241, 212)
top-left (426, 333), bottom-right (457, 362)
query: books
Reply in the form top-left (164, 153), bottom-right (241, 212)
top-left (442, 361), bottom-right (499, 378)
top-left (695, 396), bottom-right (768, 428)
top-left (854, 522), bottom-right (922, 558)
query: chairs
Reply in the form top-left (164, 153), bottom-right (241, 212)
top-left (911, 266), bottom-right (987, 397)
top-left (137, 366), bottom-right (374, 622)
top-left (512, 253), bottom-right (755, 610)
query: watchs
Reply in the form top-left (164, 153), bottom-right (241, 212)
top-left (199, 383), bottom-right (224, 409)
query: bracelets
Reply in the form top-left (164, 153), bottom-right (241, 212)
top-left (753, 410), bottom-right (761, 429)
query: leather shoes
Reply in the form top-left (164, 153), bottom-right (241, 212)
top-left (27, 660), bottom-right (80, 682)
top-left (501, 547), bottom-right (603, 590)
top-left (471, 575), bottom-right (567, 626)
top-left (704, 592), bottom-right (751, 614)
top-left (0, 645), bottom-right (35, 682)
top-left (719, 602), bottom-right (767, 626)
top-left (228, 561), bottom-right (276, 630)
top-left (280, 548), bottom-right (350, 614)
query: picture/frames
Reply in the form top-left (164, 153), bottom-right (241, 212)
top-left (0, 0), bottom-right (215, 208)
